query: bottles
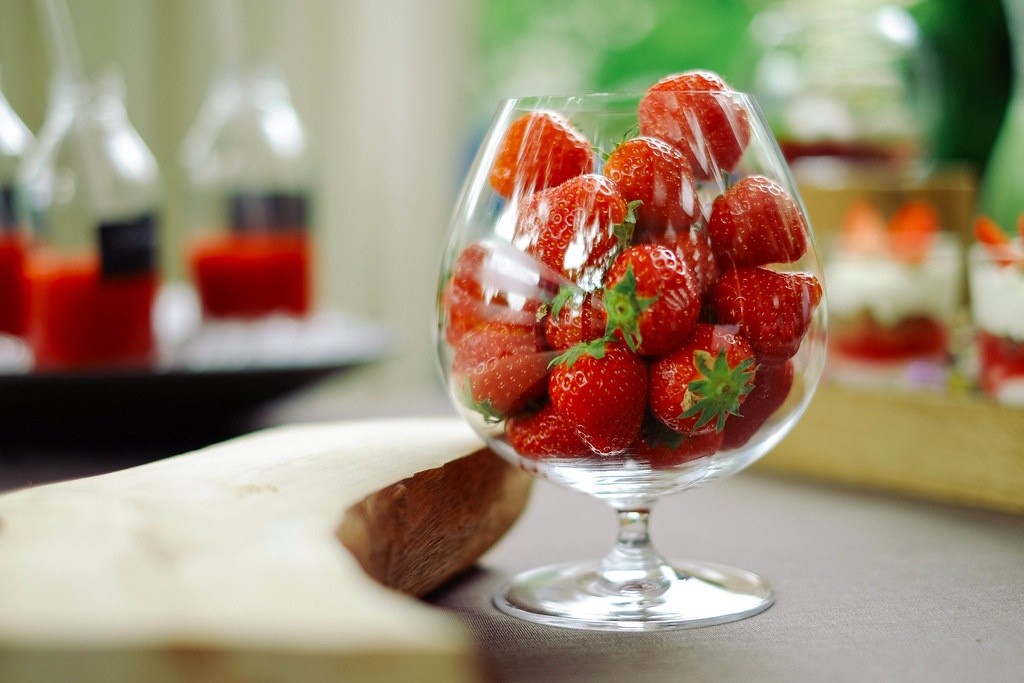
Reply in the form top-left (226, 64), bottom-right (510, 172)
top-left (183, 66), bottom-right (314, 332)
top-left (0, 64), bottom-right (174, 377)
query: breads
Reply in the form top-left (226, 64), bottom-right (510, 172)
top-left (333, 431), bottom-right (535, 597)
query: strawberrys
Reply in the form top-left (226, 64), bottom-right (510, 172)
top-left (445, 68), bottom-right (824, 471)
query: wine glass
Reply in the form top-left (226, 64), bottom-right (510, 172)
top-left (431, 88), bottom-right (830, 632)
